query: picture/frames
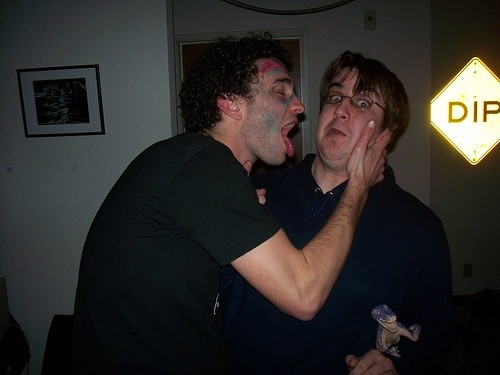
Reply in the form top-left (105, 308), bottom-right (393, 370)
top-left (15, 63), bottom-right (106, 139)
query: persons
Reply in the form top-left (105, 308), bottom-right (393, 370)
top-left (74, 31), bottom-right (393, 375)
top-left (217, 51), bottom-right (453, 375)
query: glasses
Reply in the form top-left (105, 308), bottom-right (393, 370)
top-left (321, 91), bottom-right (386, 112)
